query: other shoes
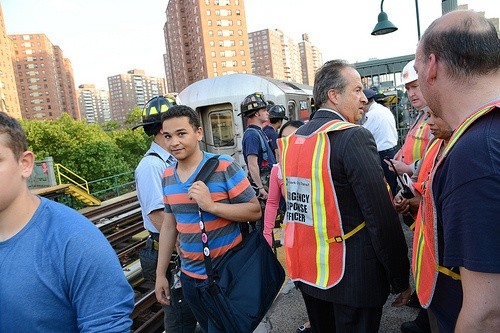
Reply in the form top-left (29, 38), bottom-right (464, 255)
top-left (405, 295), bottom-right (421, 308)
top-left (401, 320), bottom-right (432, 333)
top-left (296, 325), bottom-right (311, 333)
top-left (272, 240), bottom-right (282, 247)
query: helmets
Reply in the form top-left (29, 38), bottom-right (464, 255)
top-left (238, 91), bottom-right (273, 119)
top-left (363, 89), bottom-right (375, 98)
top-left (268, 104), bottom-right (289, 120)
top-left (401, 59), bottom-right (418, 85)
top-left (131, 94), bottom-right (178, 132)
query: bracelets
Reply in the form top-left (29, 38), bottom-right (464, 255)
top-left (258, 186), bottom-right (264, 189)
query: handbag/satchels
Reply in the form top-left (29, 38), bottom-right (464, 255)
top-left (195, 153), bottom-right (286, 333)
top-left (138, 151), bottom-right (174, 286)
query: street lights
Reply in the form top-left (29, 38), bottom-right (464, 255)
top-left (370, 0), bottom-right (421, 42)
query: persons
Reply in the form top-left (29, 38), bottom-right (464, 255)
top-left (394, 107), bottom-right (453, 333)
top-left (131, 95), bottom-right (203, 333)
top-left (410, 9), bottom-right (500, 333)
top-left (384, 60), bottom-right (434, 309)
top-left (155, 105), bottom-right (262, 333)
top-left (0, 110), bottom-right (135, 333)
top-left (237, 62), bottom-right (398, 333)
top-left (276, 59), bottom-right (412, 333)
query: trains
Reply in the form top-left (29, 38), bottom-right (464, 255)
top-left (175, 73), bottom-right (315, 179)
